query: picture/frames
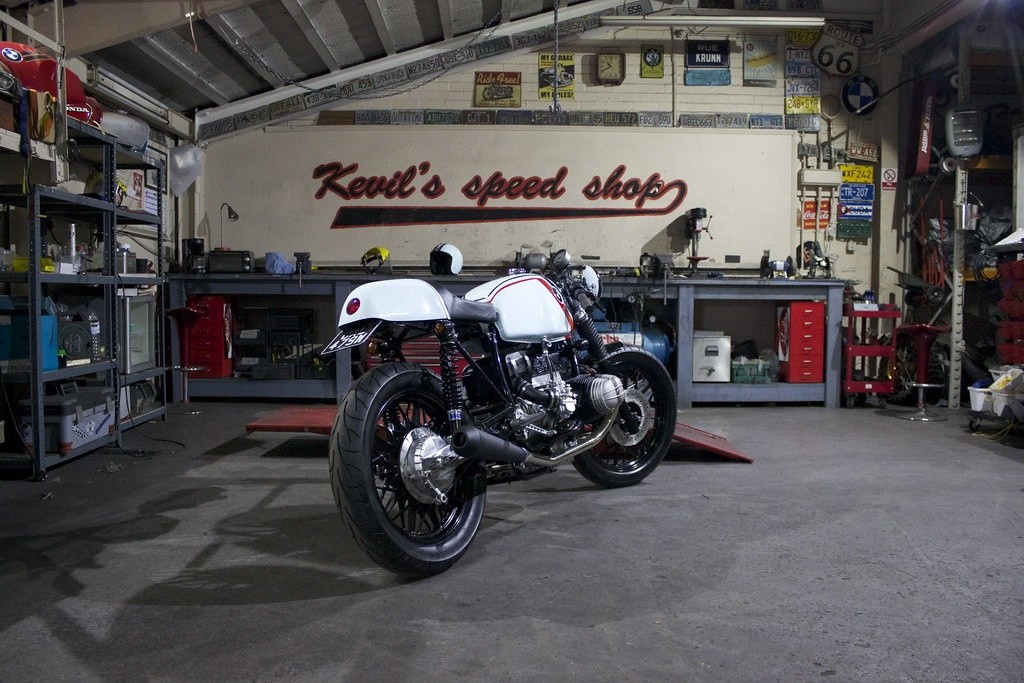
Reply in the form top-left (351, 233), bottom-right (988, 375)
top-left (144, 145), bottom-right (168, 196)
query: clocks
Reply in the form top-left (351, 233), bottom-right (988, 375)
top-left (597, 52), bottom-right (626, 86)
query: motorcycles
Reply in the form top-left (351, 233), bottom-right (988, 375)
top-left (320, 242), bottom-right (685, 583)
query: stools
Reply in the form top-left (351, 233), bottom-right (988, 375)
top-left (164, 306), bottom-right (207, 416)
top-left (893, 323), bottom-right (952, 421)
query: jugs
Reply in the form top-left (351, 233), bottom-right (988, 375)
top-left (190, 255), bottom-right (205, 270)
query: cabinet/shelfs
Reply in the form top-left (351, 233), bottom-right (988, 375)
top-left (915, 51), bottom-right (1023, 282)
top-left (188, 296), bottom-right (233, 378)
top-left (843, 301), bottom-right (903, 409)
top-left (110, 143), bottom-right (168, 430)
top-left (0, 118), bottom-right (123, 480)
top-left (773, 301), bottom-right (824, 383)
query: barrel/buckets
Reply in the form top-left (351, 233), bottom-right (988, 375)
top-left (953, 191), bottom-right (983, 232)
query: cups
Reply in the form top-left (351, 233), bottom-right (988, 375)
top-left (118, 243), bottom-right (129, 252)
top-left (136, 259), bottom-right (153, 273)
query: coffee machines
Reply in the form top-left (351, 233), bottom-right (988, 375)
top-left (182, 238), bottom-right (206, 273)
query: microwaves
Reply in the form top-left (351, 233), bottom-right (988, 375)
top-left (208, 250), bottom-right (255, 273)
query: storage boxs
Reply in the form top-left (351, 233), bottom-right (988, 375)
top-left (0, 295), bottom-right (58, 373)
top-left (990, 368), bottom-right (1024, 394)
top-left (990, 390), bottom-right (1024, 416)
top-left (693, 335), bottom-right (731, 383)
top-left (968, 387), bottom-right (992, 411)
top-left (732, 363), bottom-right (770, 384)
top-left (15, 385), bottom-right (116, 456)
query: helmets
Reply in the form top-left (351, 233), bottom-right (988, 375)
top-left (430, 242), bottom-right (463, 274)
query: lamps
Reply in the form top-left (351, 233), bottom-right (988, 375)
top-left (599, 0), bottom-right (826, 29)
top-left (220, 203), bottom-right (239, 248)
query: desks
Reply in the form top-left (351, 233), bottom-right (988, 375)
top-left (165, 272), bottom-right (847, 409)
